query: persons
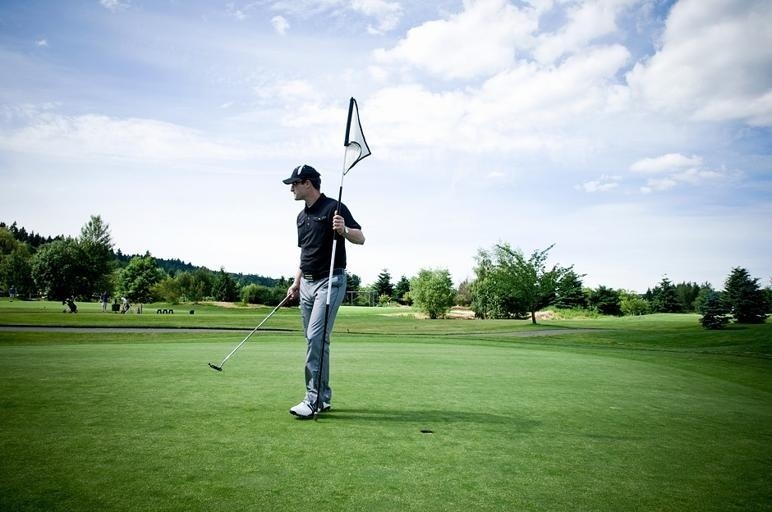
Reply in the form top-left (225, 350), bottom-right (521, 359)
top-left (9, 284), bottom-right (16, 302)
top-left (283, 165), bottom-right (365, 418)
top-left (120, 296), bottom-right (129, 313)
top-left (101, 291), bottom-right (108, 311)
top-left (62, 299), bottom-right (77, 313)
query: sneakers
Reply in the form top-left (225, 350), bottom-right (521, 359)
top-left (290, 400), bottom-right (331, 417)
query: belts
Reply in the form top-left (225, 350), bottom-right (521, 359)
top-left (303, 269), bottom-right (343, 282)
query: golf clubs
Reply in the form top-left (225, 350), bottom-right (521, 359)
top-left (207, 290), bottom-right (294, 372)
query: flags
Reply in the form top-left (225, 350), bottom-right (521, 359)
top-left (342, 95), bottom-right (372, 172)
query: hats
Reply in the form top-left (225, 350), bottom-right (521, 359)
top-left (282, 164), bottom-right (321, 184)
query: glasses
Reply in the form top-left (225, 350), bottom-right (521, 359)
top-left (293, 182), bottom-right (300, 186)
